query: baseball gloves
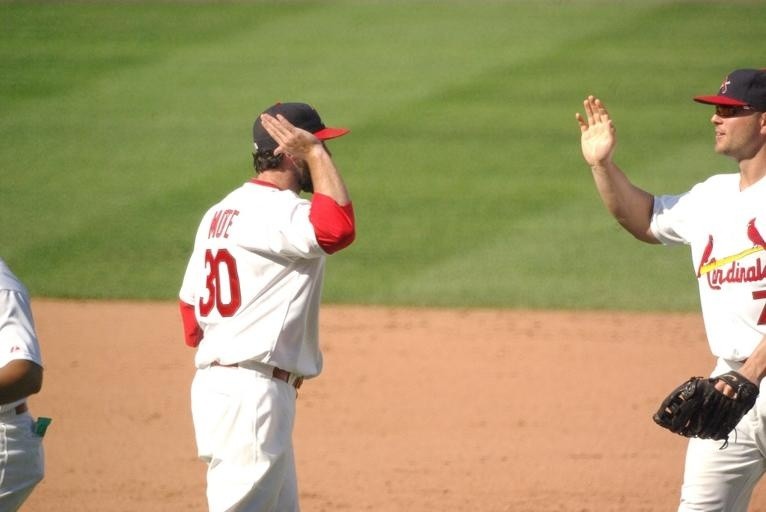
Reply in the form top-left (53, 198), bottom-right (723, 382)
top-left (652, 370), bottom-right (760, 441)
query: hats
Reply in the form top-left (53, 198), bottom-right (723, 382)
top-left (692, 68), bottom-right (766, 112)
top-left (252, 103), bottom-right (350, 151)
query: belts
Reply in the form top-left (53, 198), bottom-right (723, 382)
top-left (210, 361), bottom-right (304, 389)
top-left (14, 402), bottom-right (28, 415)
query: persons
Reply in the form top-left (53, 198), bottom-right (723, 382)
top-left (574, 68), bottom-right (766, 512)
top-left (0, 257), bottom-right (46, 512)
top-left (177, 100), bottom-right (357, 512)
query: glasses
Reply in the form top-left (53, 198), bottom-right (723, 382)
top-left (716, 105), bottom-right (760, 118)
